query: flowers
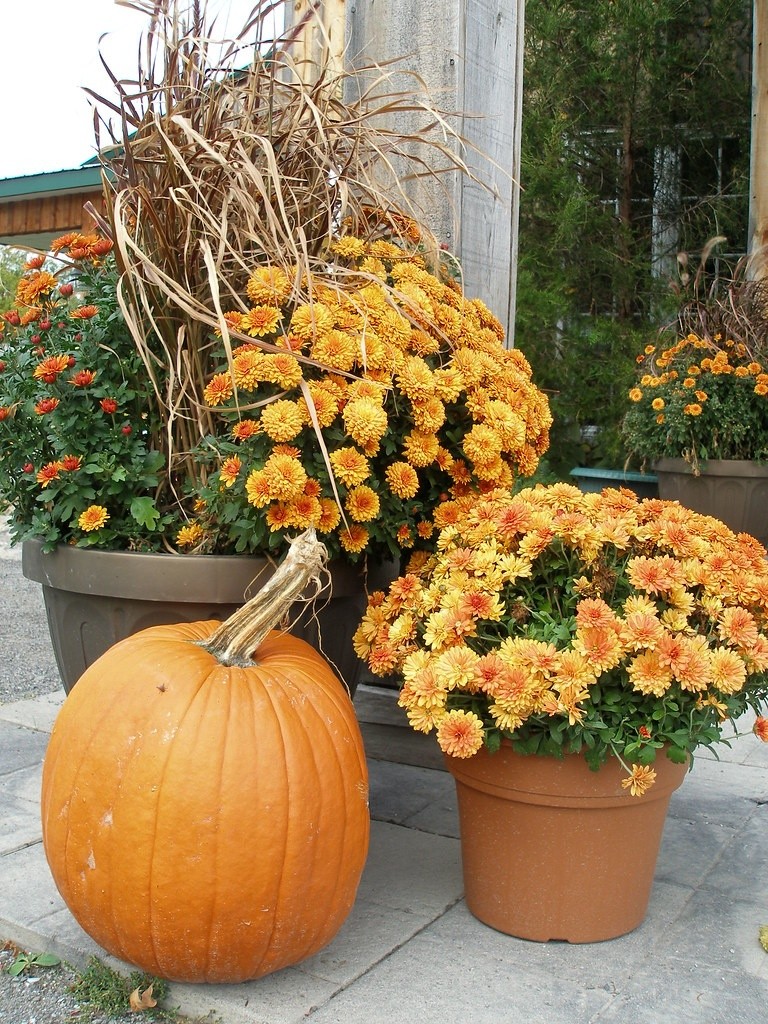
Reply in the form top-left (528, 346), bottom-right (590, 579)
top-left (0, 0), bottom-right (554, 553)
top-left (353, 483), bottom-right (768, 797)
top-left (616, 235), bottom-right (767, 474)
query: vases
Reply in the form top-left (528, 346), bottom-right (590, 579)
top-left (652, 458), bottom-right (767, 550)
top-left (442, 737), bottom-right (687, 943)
top-left (24, 534), bottom-right (361, 701)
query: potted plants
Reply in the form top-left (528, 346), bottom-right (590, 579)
top-left (569, 330), bottom-right (658, 498)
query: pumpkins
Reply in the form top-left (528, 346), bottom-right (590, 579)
top-left (41, 530), bottom-right (371, 985)
